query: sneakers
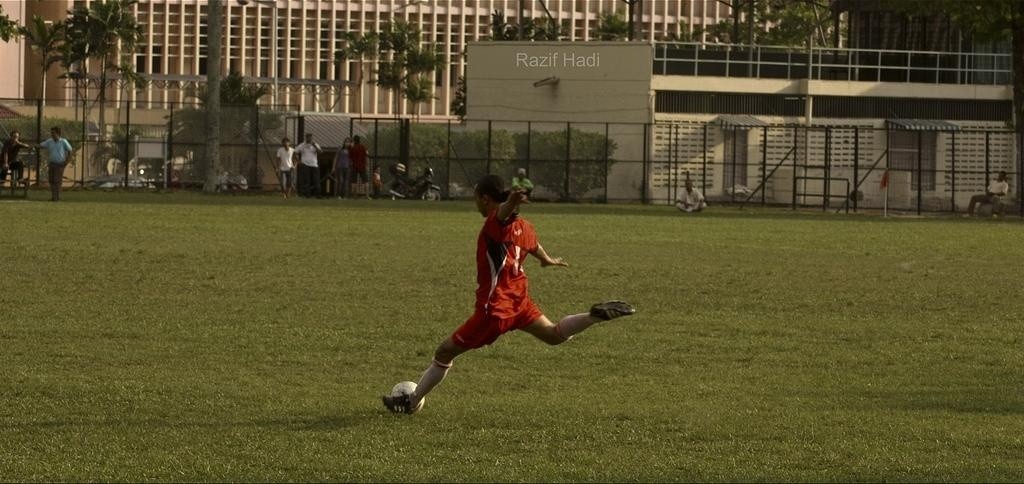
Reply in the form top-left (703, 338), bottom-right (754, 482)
top-left (588, 300), bottom-right (635, 323)
top-left (381, 393), bottom-right (418, 416)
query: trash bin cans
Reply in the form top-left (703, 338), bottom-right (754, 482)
top-left (887, 170), bottom-right (911, 210)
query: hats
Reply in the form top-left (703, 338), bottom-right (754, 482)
top-left (518, 168), bottom-right (527, 176)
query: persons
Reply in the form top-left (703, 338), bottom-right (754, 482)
top-left (275, 135), bottom-right (299, 201)
top-left (370, 164), bottom-right (383, 198)
top-left (959, 169), bottom-right (1009, 222)
top-left (349, 133), bottom-right (373, 201)
top-left (294, 131), bottom-right (324, 197)
top-left (511, 168), bottom-right (534, 204)
top-left (674, 177), bottom-right (713, 216)
top-left (330, 136), bottom-right (353, 200)
top-left (0, 130), bottom-right (34, 188)
top-left (379, 171), bottom-right (636, 416)
top-left (33, 124), bottom-right (73, 202)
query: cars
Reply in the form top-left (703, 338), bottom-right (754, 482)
top-left (72, 175), bottom-right (155, 192)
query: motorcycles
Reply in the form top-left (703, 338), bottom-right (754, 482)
top-left (387, 162), bottom-right (441, 202)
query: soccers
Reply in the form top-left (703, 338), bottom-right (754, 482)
top-left (391, 381), bottom-right (425, 414)
